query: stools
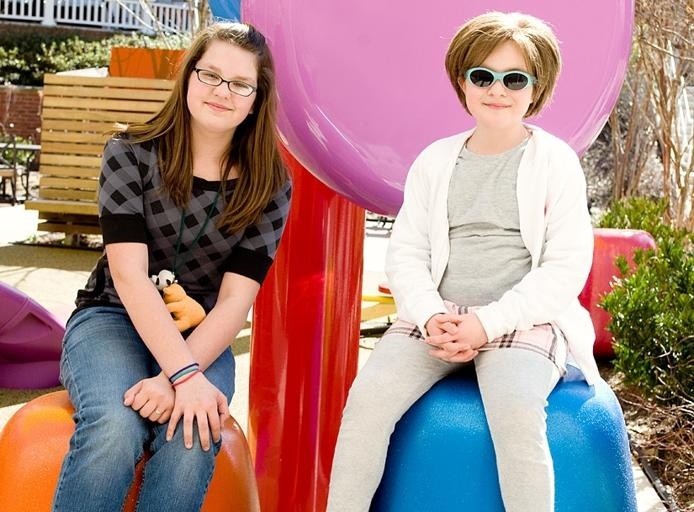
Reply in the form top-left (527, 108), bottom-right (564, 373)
top-left (369, 371), bottom-right (637, 512)
top-left (2, 389), bottom-right (260, 511)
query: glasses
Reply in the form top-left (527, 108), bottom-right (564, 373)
top-left (193, 67), bottom-right (257, 97)
top-left (464, 67), bottom-right (538, 90)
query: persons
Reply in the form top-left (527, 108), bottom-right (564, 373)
top-left (51, 20), bottom-right (293, 512)
top-left (324, 9), bottom-right (601, 512)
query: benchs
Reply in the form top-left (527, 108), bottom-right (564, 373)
top-left (23, 199), bottom-right (100, 248)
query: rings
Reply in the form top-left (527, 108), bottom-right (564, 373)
top-left (155, 409), bottom-right (163, 416)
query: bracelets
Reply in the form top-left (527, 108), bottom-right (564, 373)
top-left (169, 363), bottom-right (200, 386)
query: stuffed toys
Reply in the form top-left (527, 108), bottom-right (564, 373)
top-left (160, 283), bottom-right (207, 332)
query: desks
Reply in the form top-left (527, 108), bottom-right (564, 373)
top-left (0, 143), bottom-right (40, 200)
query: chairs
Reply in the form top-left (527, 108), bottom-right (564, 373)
top-left (0, 129), bottom-right (18, 207)
